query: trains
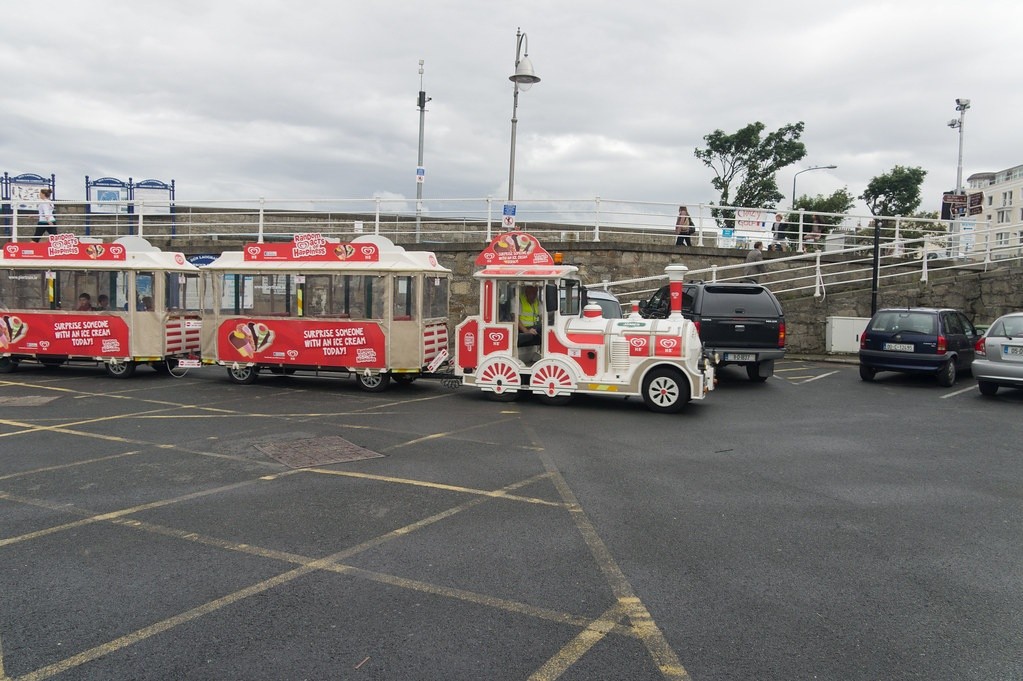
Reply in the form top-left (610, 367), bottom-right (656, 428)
top-left (0, 232), bottom-right (718, 410)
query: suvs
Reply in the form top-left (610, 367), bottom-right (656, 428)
top-left (500, 288), bottom-right (625, 319)
top-left (637, 276), bottom-right (789, 383)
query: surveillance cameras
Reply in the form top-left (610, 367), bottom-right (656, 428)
top-left (947, 119), bottom-right (957, 126)
top-left (956, 98), bottom-right (971, 107)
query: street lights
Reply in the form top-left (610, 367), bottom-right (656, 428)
top-left (946, 97), bottom-right (971, 265)
top-left (504, 26), bottom-right (543, 235)
top-left (790, 163), bottom-right (839, 248)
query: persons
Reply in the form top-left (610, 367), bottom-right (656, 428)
top-left (31, 189), bottom-right (57, 242)
top-left (744, 242), bottom-right (769, 283)
top-left (804, 215), bottom-right (822, 251)
top-left (124, 288), bottom-right (145, 311)
top-left (892, 234), bottom-right (905, 258)
top-left (675, 207), bottom-right (694, 246)
top-left (767, 214), bottom-right (788, 252)
top-left (499, 286), bottom-right (543, 346)
top-left (95, 294), bottom-right (111, 311)
top-left (77, 293), bottom-right (91, 311)
top-left (914, 243), bottom-right (923, 259)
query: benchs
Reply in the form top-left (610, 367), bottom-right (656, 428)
top-left (499, 303), bottom-right (542, 346)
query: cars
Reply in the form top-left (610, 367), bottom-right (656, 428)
top-left (857, 307), bottom-right (985, 388)
top-left (971, 311), bottom-right (1023, 396)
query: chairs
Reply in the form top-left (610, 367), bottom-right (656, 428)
top-left (896, 318), bottom-right (915, 330)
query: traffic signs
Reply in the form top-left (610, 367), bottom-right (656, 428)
top-left (949, 203), bottom-right (966, 215)
top-left (970, 192), bottom-right (984, 206)
top-left (942, 193), bottom-right (967, 204)
top-left (969, 206), bottom-right (983, 215)
